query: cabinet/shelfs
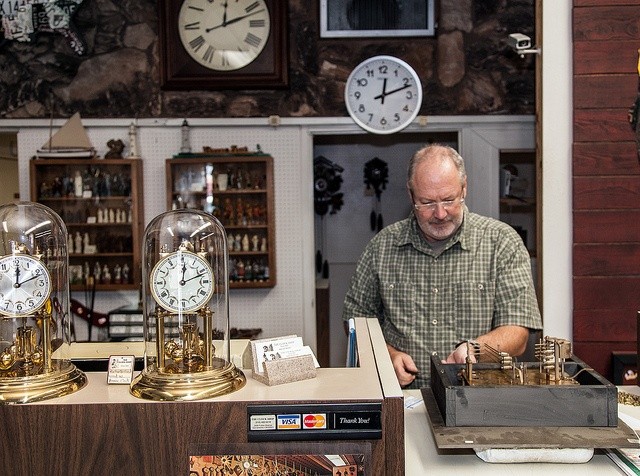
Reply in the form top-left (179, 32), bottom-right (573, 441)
top-left (0, 316), bottom-right (403, 475)
top-left (28, 157), bottom-right (145, 292)
top-left (165, 153), bottom-right (277, 291)
top-left (497, 147), bottom-right (535, 261)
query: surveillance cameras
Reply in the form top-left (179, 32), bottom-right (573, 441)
top-left (507, 32), bottom-right (530, 58)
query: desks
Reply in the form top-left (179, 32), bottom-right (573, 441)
top-left (50, 339), bottom-right (254, 372)
top-left (401, 384), bottom-right (636, 476)
top-left (315, 278), bottom-right (331, 368)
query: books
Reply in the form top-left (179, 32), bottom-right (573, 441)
top-left (35, 147), bottom-right (97, 158)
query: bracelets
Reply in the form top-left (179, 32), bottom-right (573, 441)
top-left (452, 339), bottom-right (482, 363)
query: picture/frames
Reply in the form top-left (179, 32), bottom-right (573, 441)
top-left (610, 350), bottom-right (638, 386)
top-left (317, 1), bottom-right (442, 41)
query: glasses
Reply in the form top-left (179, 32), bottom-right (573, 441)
top-left (410, 189), bottom-right (465, 211)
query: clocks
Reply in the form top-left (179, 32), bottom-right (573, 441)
top-left (129, 208), bottom-right (246, 401)
top-left (345, 56), bottom-right (423, 136)
top-left (0, 202), bottom-right (89, 404)
top-left (159, 0), bottom-right (290, 92)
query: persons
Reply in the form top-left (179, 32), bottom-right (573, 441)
top-left (341, 141), bottom-right (543, 385)
